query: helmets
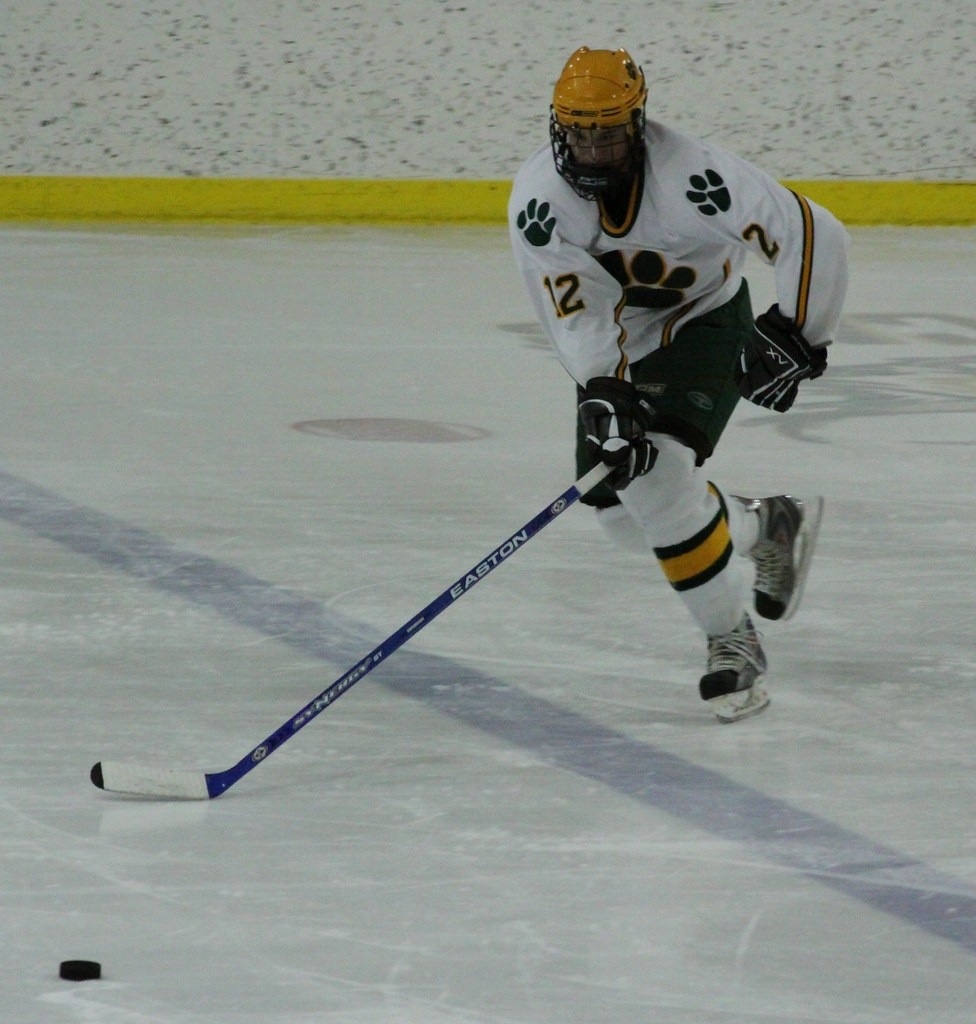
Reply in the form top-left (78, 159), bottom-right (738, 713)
top-left (546, 46), bottom-right (648, 202)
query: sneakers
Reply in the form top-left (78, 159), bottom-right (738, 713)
top-left (698, 611), bottom-right (769, 723)
top-left (731, 493), bottom-right (824, 622)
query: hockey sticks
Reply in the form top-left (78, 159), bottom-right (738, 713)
top-left (89, 430), bottom-right (648, 802)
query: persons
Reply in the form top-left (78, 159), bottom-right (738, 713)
top-left (508, 47), bottom-right (848, 724)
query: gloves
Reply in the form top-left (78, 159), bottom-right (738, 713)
top-left (576, 377), bottom-right (660, 491)
top-left (734, 303), bottom-right (832, 414)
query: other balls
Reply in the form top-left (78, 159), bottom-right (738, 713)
top-left (58, 960), bottom-right (102, 981)
